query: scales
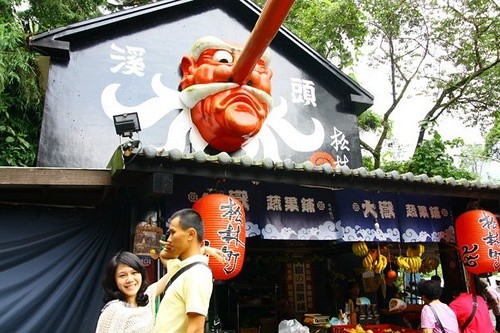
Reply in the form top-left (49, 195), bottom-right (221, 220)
top-left (304, 314), bottom-right (330, 325)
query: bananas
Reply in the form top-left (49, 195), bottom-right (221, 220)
top-left (374, 254), bottom-right (387, 273)
top-left (362, 254), bottom-right (373, 271)
top-left (397, 244), bottom-right (425, 273)
top-left (352, 241), bottom-right (369, 257)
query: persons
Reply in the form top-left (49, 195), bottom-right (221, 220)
top-left (443, 283), bottom-right (495, 333)
top-left (480, 281), bottom-right (500, 333)
top-left (417, 280), bottom-right (459, 333)
top-left (155, 208), bottom-right (213, 333)
top-left (179, 36), bottom-right (273, 153)
top-left (350, 282), bottom-right (362, 309)
top-left (95, 246), bottom-right (230, 333)
top-left (431, 275), bottom-right (445, 304)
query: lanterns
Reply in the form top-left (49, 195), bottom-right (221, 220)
top-left (454, 210), bottom-right (500, 278)
top-left (193, 193), bottom-right (247, 280)
